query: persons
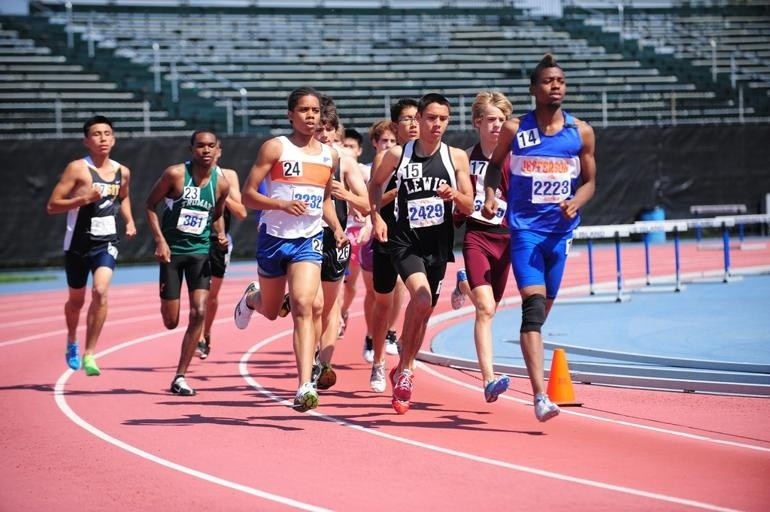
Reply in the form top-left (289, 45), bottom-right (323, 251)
top-left (450, 91), bottom-right (514, 404)
top-left (194, 137), bottom-right (248, 360)
top-left (480, 56), bottom-right (596, 423)
top-left (144, 128), bottom-right (231, 396)
top-left (368, 93), bottom-right (475, 416)
top-left (234, 86), bottom-right (349, 413)
top-left (47, 115), bottom-right (137, 377)
top-left (338, 127), bottom-right (363, 338)
top-left (279, 96), bottom-right (371, 390)
top-left (359, 121), bottom-right (407, 362)
top-left (365, 99), bottom-right (420, 392)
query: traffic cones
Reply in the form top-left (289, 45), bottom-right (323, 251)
top-left (546, 349), bottom-right (584, 405)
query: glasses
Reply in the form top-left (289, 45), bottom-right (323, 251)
top-left (400, 117), bottom-right (419, 125)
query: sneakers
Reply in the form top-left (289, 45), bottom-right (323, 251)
top-left (81, 353), bottom-right (101, 376)
top-left (338, 313), bottom-right (348, 338)
top-left (235, 281), bottom-right (259, 330)
top-left (370, 360), bottom-right (386, 393)
top-left (66, 341), bottom-right (80, 370)
top-left (279, 293), bottom-right (290, 317)
top-left (484, 376), bottom-right (510, 402)
top-left (535, 394), bottom-right (560, 421)
top-left (170, 375), bottom-right (195, 396)
top-left (390, 368), bottom-right (410, 414)
top-left (451, 268), bottom-right (467, 309)
top-left (390, 367), bottom-right (413, 400)
top-left (384, 330), bottom-right (398, 355)
top-left (193, 341), bottom-right (205, 357)
top-left (201, 346), bottom-right (210, 359)
top-left (363, 336), bottom-right (374, 362)
top-left (292, 383), bottom-right (319, 413)
top-left (315, 362), bottom-right (336, 390)
